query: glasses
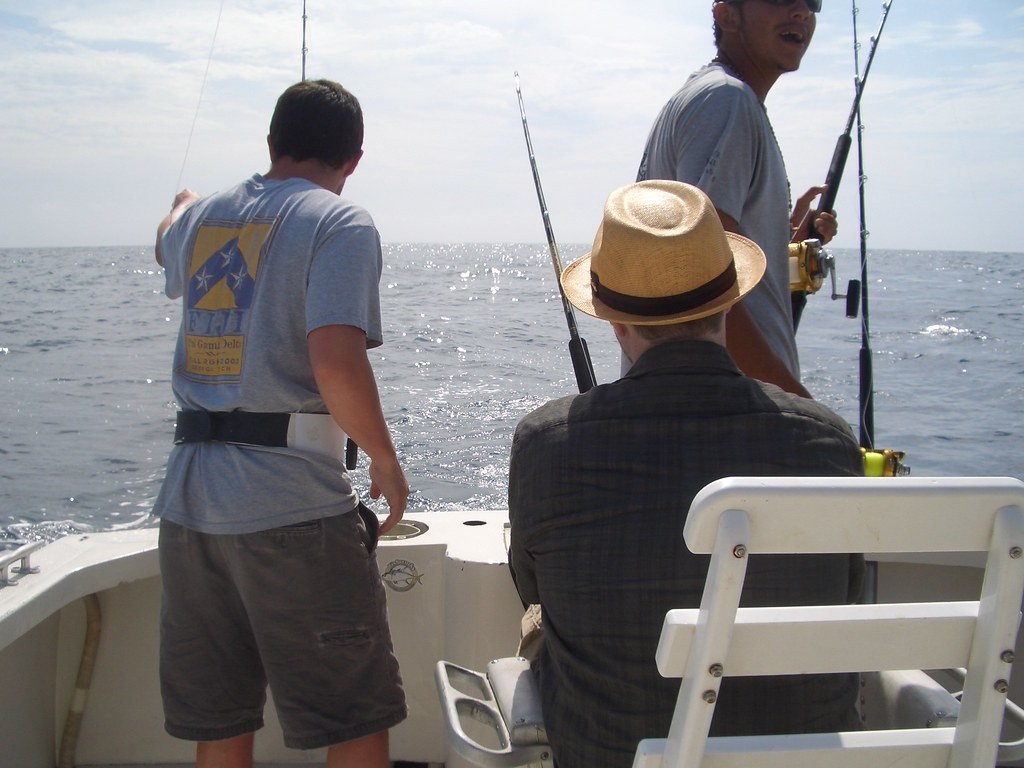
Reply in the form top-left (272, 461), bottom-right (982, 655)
top-left (767, 0), bottom-right (822, 13)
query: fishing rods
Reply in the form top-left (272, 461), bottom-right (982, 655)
top-left (510, 63), bottom-right (600, 396)
top-left (849, 0), bottom-right (918, 479)
top-left (787, 1), bottom-right (896, 335)
top-left (300, 1), bottom-right (359, 482)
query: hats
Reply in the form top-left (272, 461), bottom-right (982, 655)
top-left (560, 179), bottom-right (767, 325)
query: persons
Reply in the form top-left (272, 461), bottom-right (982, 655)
top-left (510, 180), bottom-right (865, 768)
top-left (156, 79), bottom-right (409, 768)
top-left (621, 0), bottom-right (837, 398)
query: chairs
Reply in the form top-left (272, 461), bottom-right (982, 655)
top-left (436, 474), bottom-right (1024, 768)
